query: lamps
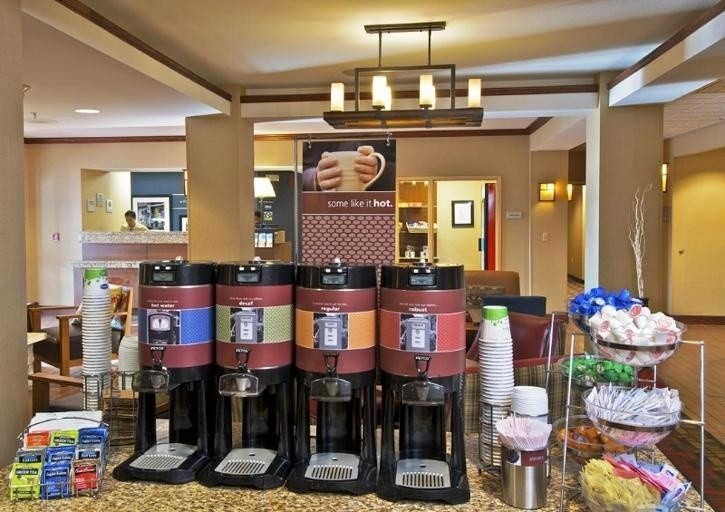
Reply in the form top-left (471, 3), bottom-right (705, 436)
top-left (661, 161), bottom-right (669, 192)
top-left (323, 21), bottom-right (485, 129)
top-left (538, 183), bottom-right (556, 201)
top-left (254, 177), bottom-right (276, 227)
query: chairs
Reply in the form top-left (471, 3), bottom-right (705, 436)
top-left (27, 282), bottom-right (134, 416)
top-left (461, 270), bottom-right (638, 431)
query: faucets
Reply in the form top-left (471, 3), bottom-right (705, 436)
top-left (414, 354), bottom-right (430, 400)
top-left (148, 346), bottom-right (164, 388)
top-left (234, 349), bottom-right (251, 392)
top-left (323, 352), bottom-right (340, 396)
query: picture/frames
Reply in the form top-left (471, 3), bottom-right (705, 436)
top-left (131, 194), bottom-right (172, 232)
top-left (451, 200), bottom-right (474, 228)
top-left (179, 215), bottom-right (188, 231)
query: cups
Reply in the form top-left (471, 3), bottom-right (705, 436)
top-left (405, 246), bottom-right (426, 258)
top-left (477, 304), bottom-right (550, 469)
top-left (498, 445), bottom-right (552, 510)
top-left (321, 149), bottom-right (386, 191)
top-left (79, 266), bottom-right (141, 412)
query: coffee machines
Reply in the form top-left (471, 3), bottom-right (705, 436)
top-left (212, 259), bottom-right (293, 489)
top-left (378, 261), bottom-right (474, 503)
top-left (283, 257), bottom-right (379, 497)
top-left (112, 257), bottom-right (212, 484)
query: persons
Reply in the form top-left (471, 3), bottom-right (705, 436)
top-left (302, 140), bottom-right (395, 192)
top-left (120, 210), bottom-right (149, 232)
top-left (254, 210), bottom-right (272, 229)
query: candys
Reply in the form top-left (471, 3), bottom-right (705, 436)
top-left (557, 287), bottom-right (682, 451)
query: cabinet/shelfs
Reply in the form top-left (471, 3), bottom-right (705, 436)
top-left (397, 181), bottom-right (436, 263)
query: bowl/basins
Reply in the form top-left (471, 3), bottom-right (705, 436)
top-left (576, 456), bottom-right (686, 512)
top-left (582, 383), bottom-right (685, 450)
top-left (553, 413), bottom-right (635, 465)
top-left (556, 356), bottom-right (634, 394)
top-left (568, 291), bottom-right (649, 331)
top-left (583, 312), bottom-right (688, 369)
